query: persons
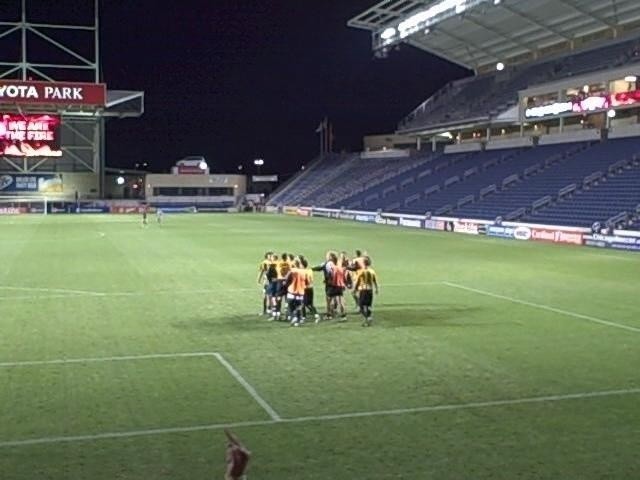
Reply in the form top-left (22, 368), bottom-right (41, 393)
top-left (142, 203), bottom-right (151, 223)
top-left (223, 425), bottom-right (250, 480)
top-left (258, 249), bottom-right (380, 328)
top-left (156, 206), bottom-right (163, 223)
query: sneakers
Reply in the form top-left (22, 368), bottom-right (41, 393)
top-left (259, 306), bottom-right (373, 328)
